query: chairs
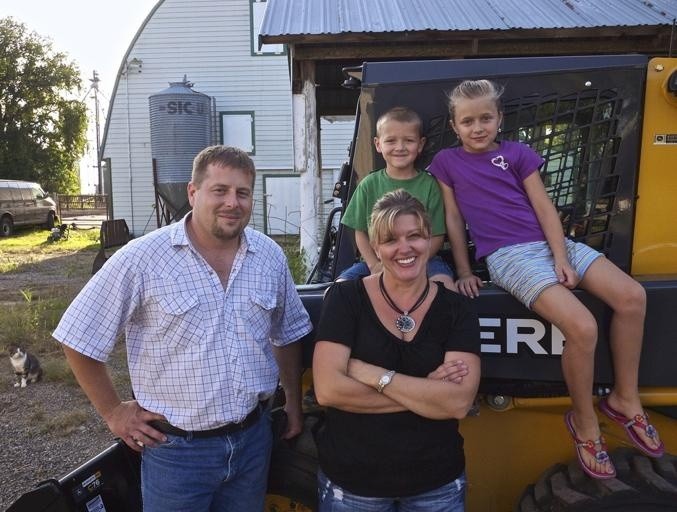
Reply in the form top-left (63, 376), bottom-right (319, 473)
top-left (99, 218), bottom-right (131, 259)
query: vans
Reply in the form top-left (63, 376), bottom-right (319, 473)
top-left (0, 179), bottom-right (58, 238)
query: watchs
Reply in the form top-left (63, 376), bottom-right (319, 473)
top-left (376, 370), bottom-right (396, 393)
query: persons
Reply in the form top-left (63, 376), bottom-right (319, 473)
top-left (427, 80), bottom-right (664, 479)
top-left (51, 145), bottom-right (315, 512)
top-left (311, 188), bottom-right (482, 510)
top-left (322, 106), bottom-right (481, 419)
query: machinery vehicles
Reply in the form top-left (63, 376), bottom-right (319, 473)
top-left (3, 54), bottom-right (676, 512)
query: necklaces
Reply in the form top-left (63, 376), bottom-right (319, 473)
top-left (379, 272), bottom-right (430, 333)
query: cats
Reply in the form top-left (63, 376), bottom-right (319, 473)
top-left (7, 341), bottom-right (43, 389)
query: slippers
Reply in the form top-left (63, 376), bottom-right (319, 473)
top-left (566, 407), bottom-right (616, 480)
top-left (598, 392), bottom-right (667, 459)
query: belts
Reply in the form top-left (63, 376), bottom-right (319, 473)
top-left (145, 401), bottom-right (264, 439)
